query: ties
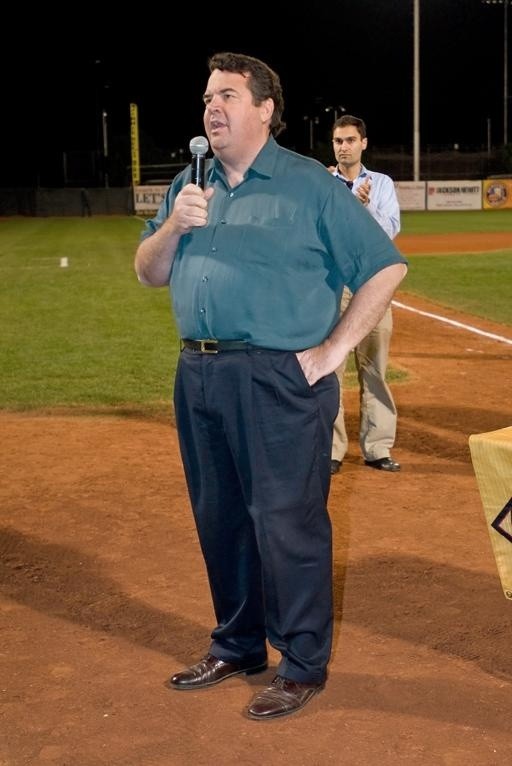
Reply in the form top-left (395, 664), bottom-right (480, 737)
top-left (346, 180), bottom-right (353, 191)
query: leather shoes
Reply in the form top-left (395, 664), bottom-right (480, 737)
top-left (241, 676), bottom-right (326, 721)
top-left (330, 460), bottom-right (341, 475)
top-left (364, 457), bottom-right (400, 472)
top-left (169, 653), bottom-right (267, 689)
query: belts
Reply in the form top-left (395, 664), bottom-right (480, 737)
top-left (182, 338), bottom-right (259, 355)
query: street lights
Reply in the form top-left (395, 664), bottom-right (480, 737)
top-left (325, 106), bottom-right (347, 124)
top-left (304, 115), bottom-right (319, 152)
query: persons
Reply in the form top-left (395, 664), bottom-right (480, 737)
top-left (81, 187), bottom-right (92, 217)
top-left (133, 50), bottom-right (409, 723)
top-left (325, 114), bottom-right (406, 475)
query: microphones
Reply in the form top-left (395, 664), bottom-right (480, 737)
top-left (187, 135), bottom-right (210, 200)
